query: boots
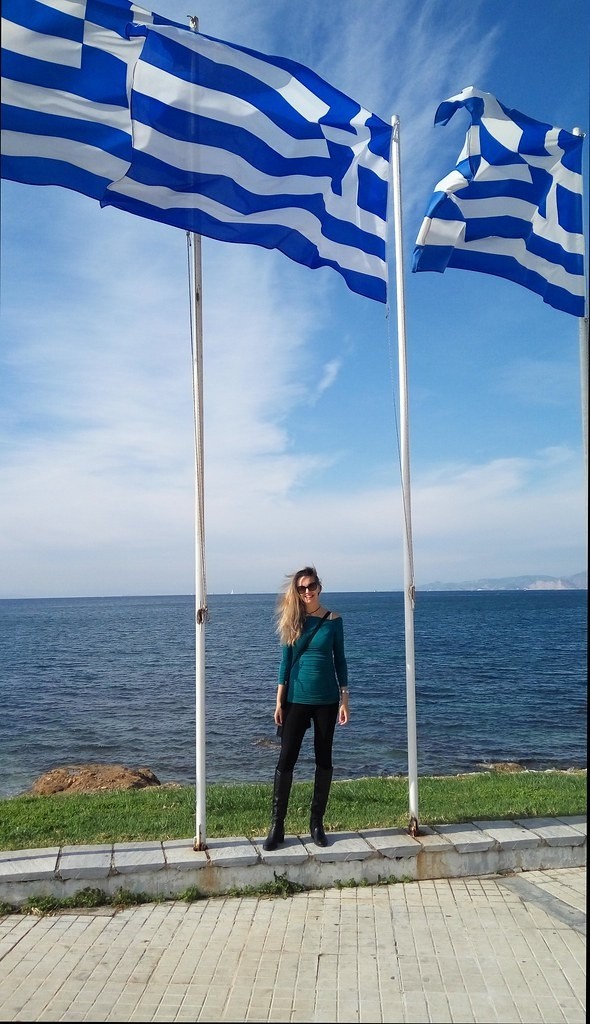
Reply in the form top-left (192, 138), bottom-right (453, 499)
top-left (310, 767), bottom-right (333, 848)
top-left (263, 773), bottom-right (293, 851)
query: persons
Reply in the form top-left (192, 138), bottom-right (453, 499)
top-left (263, 567), bottom-right (350, 851)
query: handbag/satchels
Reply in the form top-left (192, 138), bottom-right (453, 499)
top-left (276, 699), bottom-right (287, 736)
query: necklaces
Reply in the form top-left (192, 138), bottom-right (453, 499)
top-left (305, 606), bottom-right (321, 616)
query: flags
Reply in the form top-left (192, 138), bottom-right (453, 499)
top-left (410, 85), bottom-right (587, 320)
top-left (100, 23), bottom-right (395, 305)
top-left (0, 1), bottom-right (190, 200)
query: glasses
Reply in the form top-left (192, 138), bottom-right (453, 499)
top-left (296, 582), bottom-right (319, 594)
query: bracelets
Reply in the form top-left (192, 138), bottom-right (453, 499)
top-left (341, 689), bottom-right (349, 696)
